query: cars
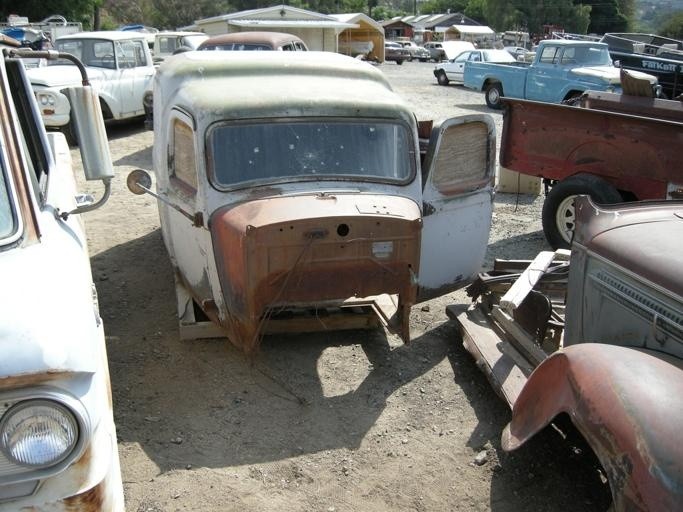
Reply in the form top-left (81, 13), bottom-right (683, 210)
top-left (384, 36), bottom-right (540, 85)
top-left (143, 30), bottom-right (310, 129)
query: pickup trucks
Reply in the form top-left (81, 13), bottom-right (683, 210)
top-left (1, 25), bottom-right (133, 512)
top-left (2, 16), bottom-right (211, 149)
top-left (462, 39), bottom-right (663, 109)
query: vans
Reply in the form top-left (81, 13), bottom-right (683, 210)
top-left (123, 49), bottom-right (497, 356)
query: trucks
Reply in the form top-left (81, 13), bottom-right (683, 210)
top-left (497, 90), bottom-right (682, 254)
